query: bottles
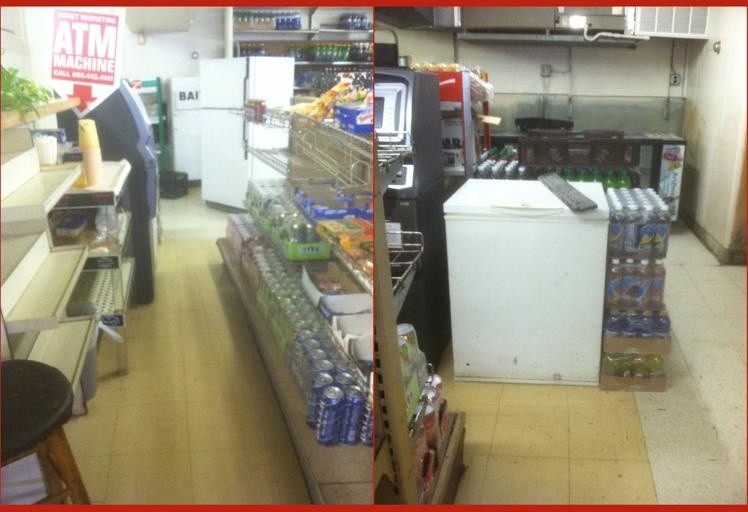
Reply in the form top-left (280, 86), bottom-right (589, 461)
top-left (477, 145), bottom-right (529, 178)
top-left (234, 10), bottom-right (374, 92)
top-left (554, 165), bottom-right (642, 187)
top-left (600, 188), bottom-right (670, 379)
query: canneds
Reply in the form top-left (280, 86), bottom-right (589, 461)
top-left (250, 211), bottom-right (374, 446)
top-left (245, 98), bottom-right (266, 122)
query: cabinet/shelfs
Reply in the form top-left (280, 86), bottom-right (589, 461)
top-left (129, 78), bottom-right (165, 172)
top-left (373, 131), bottom-right (468, 504)
top-left (215, 106), bottom-right (374, 505)
top-left (225, 7), bottom-right (374, 69)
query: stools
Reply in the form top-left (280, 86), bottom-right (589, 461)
top-left (0, 358), bottom-right (90, 504)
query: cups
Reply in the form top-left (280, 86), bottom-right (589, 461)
top-left (76, 119), bottom-right (105, 185)
top-left (34, 136), bottom-right (60, 169)
